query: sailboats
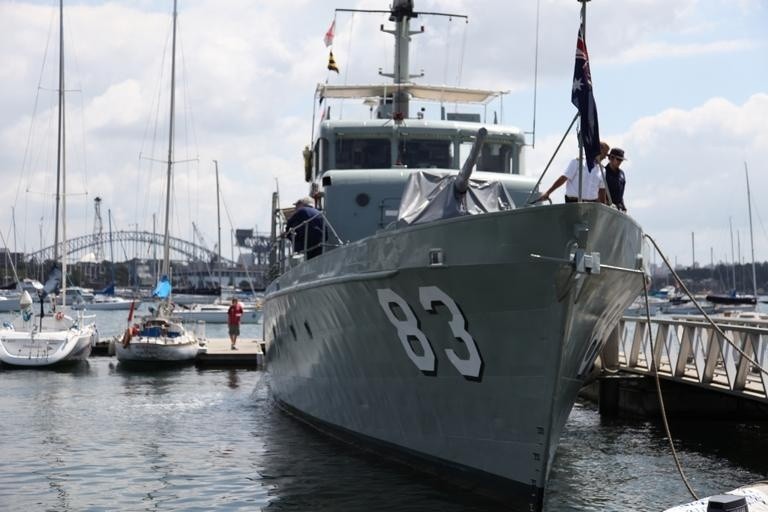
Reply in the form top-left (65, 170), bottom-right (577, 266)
top-left (623, 161), bottom-right (758, 315)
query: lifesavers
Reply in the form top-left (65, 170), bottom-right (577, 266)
top-left (122, 328), bottom-right (132, 347)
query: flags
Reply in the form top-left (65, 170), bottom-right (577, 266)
top-left (571, 3), bottom-right (600, 173)
top-left (324, 21), bottom-right (337, 47)
top-left (328, 48), bottom-right (339, 74)
top-left (127, 302), bottom-right (134, 321)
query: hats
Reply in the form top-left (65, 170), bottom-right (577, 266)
top-left (607, 148), bottom-right (627, 161)
top-left (293, 196), bottom-right (313, 208)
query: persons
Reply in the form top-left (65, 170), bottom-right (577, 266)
top-left (605, 148), bottom-right (627, 210)
top-left (51, 298), bottom-right (56, 311)
top-left (227, 299), bottom-right (243, 350)
top-left (123, 323), bottom-right (142, 348)
top-left (286, 199), bottom-right (328, 259)
top-left (538, 142), bottom-right (610, 204)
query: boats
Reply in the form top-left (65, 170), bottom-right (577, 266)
top-left (263, 0), bottom-right (651, 512)
